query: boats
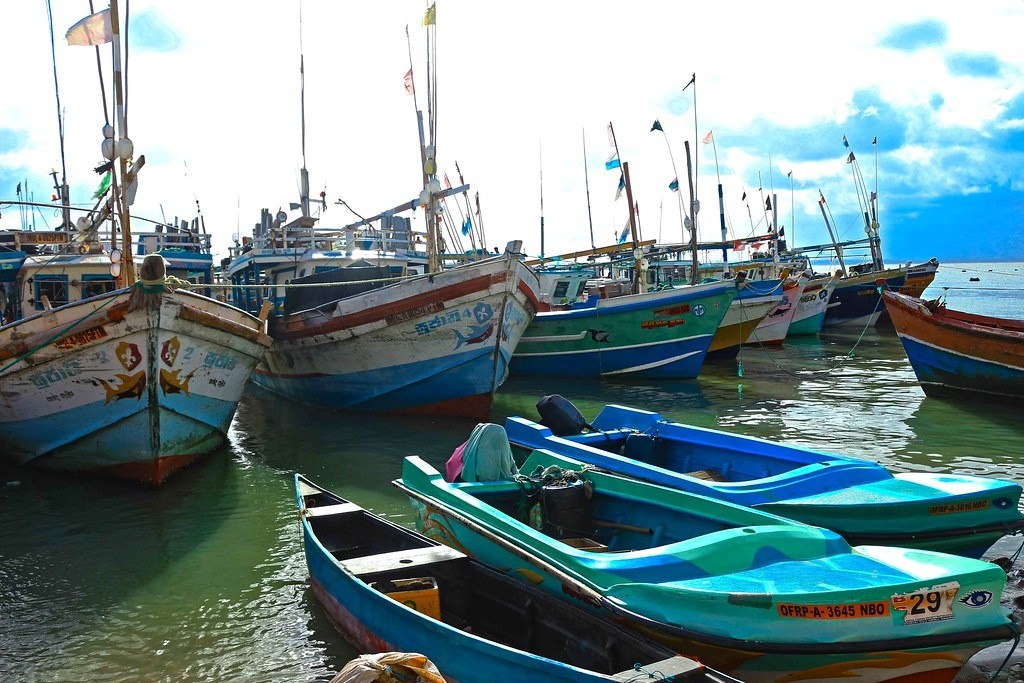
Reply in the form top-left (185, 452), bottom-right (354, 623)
top-left (0, 0), bottom-right (273, 491)
top-left (294, 471), bottom-right (748, 683)
top-left (505, 393), bottom-right (1024, 567)
top-left (497, 73), bottom-right (943, 380)
top-left (212, 0), bottom-right (542, 422)
top-left (392, 422), bottom-right (1024, 683)
top-left (873, 277), bottom-right (1024, 403)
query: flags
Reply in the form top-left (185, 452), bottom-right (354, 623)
top-left (620, 218), bottom-right (630, 243)
top-left (605, 159), bottom-right (622, 171)
top-left (403, 70), bottom-right (414, 95)
top-left (65, 8), bottom-right (113, 46)
top-left (766, 197), bottom-right (772, 210)
top-left (613, 175), bottom-right (625, 202)
top-left (843, 136), bottom-right (855, 164)
top-left (669, 178), bottom-right (678, 191)
top-left (424, 4), bottom-right (435, 24)
top-left (650, 121), bottom-right (662, 132)
top-left (702, 131), bottom-right (712, 144)
top-left (779, 228), bottom-right (784, 236)
